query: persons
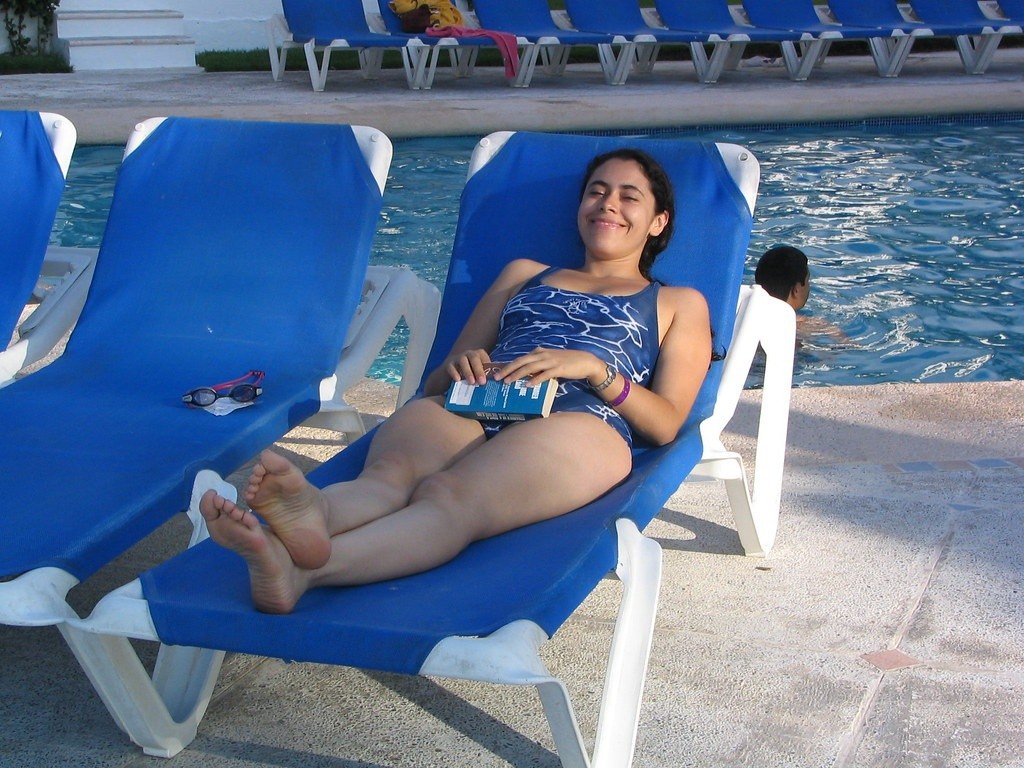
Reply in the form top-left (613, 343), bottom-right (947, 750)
top-left (199, 147), bottom-right (711, 615)
top-left (754, 246), bottom-right (859, 350)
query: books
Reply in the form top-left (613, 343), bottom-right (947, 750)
top-left (444, 363), bottom-right (558, 421)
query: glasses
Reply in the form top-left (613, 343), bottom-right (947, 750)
top-left (182, 370), bottom-right (266, 406)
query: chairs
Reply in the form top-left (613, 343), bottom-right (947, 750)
top-left (266, 1), bottom-right (1024, 94)
top-left (0, 107), bottom-right (796, 768)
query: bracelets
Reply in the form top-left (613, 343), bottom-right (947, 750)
top-left (606, 374), bottom-right (630, 406)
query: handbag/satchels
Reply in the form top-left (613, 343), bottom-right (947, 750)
top-left (401, 4), bottom-right (435, 33)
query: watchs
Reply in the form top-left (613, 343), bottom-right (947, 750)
top-left (587, 365), bottom-right (616, 393)
